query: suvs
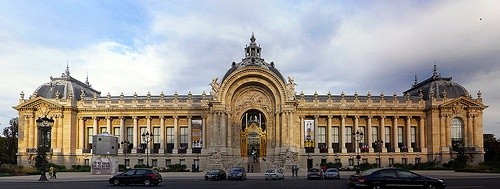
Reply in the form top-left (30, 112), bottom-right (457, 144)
top-left (228, 166), bottom-right (247, 181)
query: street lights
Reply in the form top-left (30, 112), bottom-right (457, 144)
top-left (142, 131), bottom-right (154, 167)
top-left (374, 140), bottom-right (384, 167)
top-left (304, 139), bottom-right (314, 172)
top-left (121, 140), bottom-right (131, 171)
top-left (351, 130), bottom-right (363, 173)
top-left (33, 114), bottom-right (55, 181)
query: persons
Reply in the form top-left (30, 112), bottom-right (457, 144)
top-left (322, 164), bottom-right (326, 173)
top-left (292, 165), bottom-right (298, 177)
top-left (49, 165), bottom-right (57, 179)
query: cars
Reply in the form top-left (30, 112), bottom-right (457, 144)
top-left (323, 168), bottom-right (340, 180)
top-left (306, 166), bottom-right (323, 180)
top-left (108, 167), bottom-right (163, 186)
top-left (152, 166), bottom-right (171, 172)
top-left (347, 167), bottom-right (447, 189)
top-left (204, 169), bottom-right (226, 181)
top-left (264, 169), bottom-right (285, 181)
